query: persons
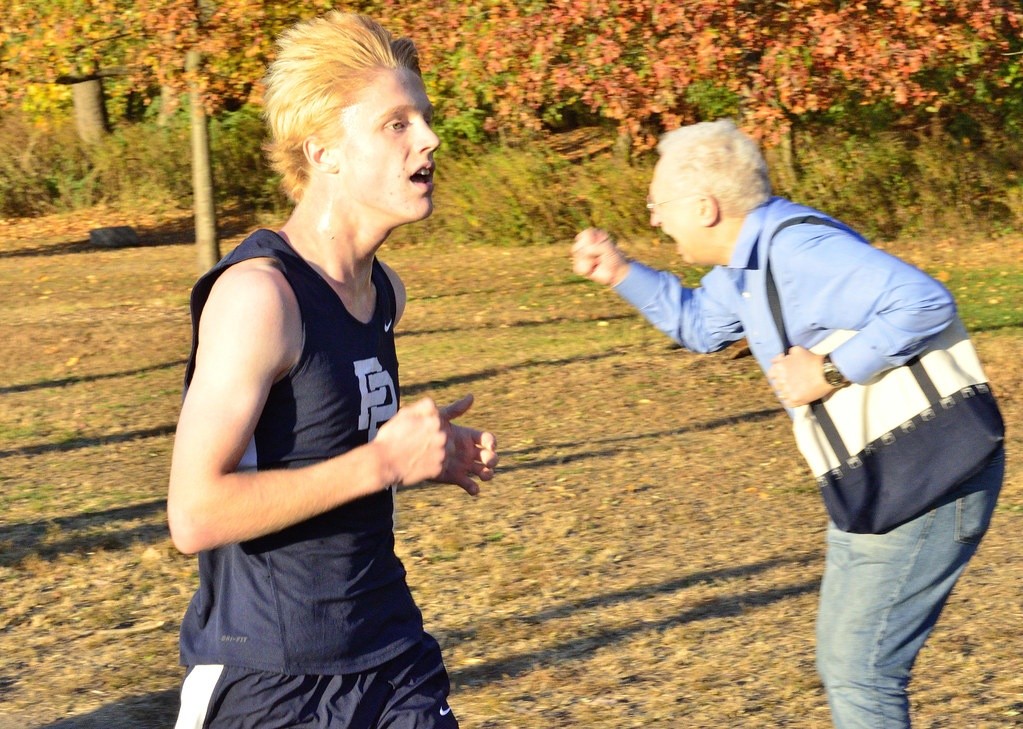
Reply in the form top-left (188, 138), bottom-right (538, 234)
top-left (160, 9), bottom-right (510, 729)
top-left (567, 118), bottom-right (1008, 729)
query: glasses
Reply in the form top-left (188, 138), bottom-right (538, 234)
top-left (646, 194), bottom-right (701, 214)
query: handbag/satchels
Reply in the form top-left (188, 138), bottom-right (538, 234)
top-left (763, 215), bottom-right (1010, 536)
